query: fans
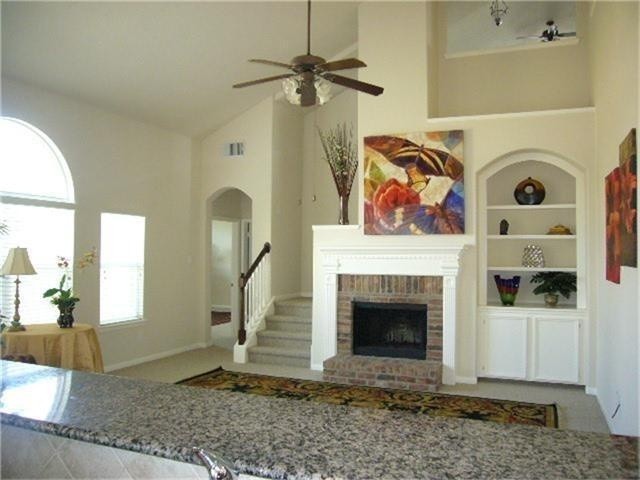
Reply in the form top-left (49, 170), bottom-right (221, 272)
top-left (232, 0), bottom-right (384, 96)
top-left (517, 21), bottom-right (576, 41)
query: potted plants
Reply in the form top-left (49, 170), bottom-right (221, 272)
top-left (315, 122), bottom-right (358, 225)
top-left (530, 270), bottom-right (577, 306)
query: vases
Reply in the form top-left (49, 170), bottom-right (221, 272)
top-left (58, 306), bottom-right (74, 329)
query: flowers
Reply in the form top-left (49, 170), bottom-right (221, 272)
top-left (42, 248), bottom-right (96, 306)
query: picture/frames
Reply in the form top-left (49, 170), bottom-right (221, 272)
top-left (619, 128), bottom-right (637, 266)
top-left (604, 167), bottom-right (620, 284)
top-left (364, 130), bottom-right (465, 235)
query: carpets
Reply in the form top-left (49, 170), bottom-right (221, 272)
top-left (174, 366), bottom-right (559, 428)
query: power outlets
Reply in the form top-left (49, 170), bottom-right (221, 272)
top-left (312, 195), bottom-right (316, 201)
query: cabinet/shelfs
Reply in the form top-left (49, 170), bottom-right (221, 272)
top-left (477, 151), bottom-right (587, 312)
top-left (479, 312), bottom-right (587, 384)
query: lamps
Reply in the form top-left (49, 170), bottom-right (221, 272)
top-left (489, 1), bottom-right (509, 26)
top-left (296, 72), bottom-right (317, 106)
top-left (0, 246), bottom-right (38, 332)
top-left (282, 74), bottom-right (333, 105)
